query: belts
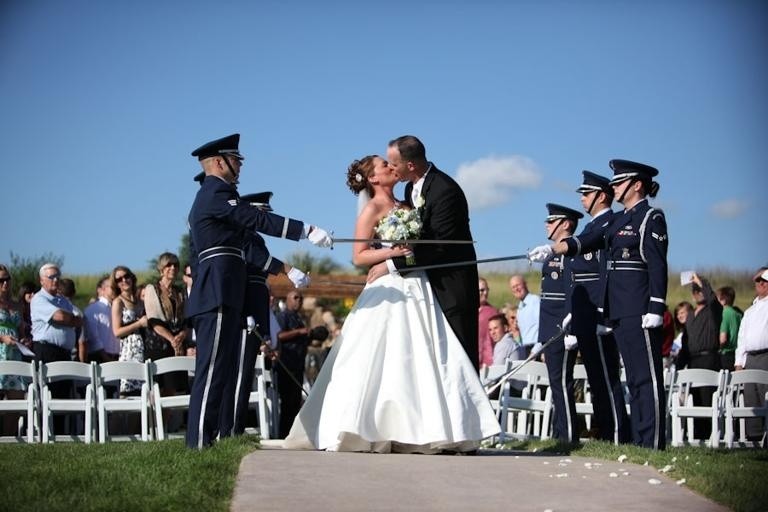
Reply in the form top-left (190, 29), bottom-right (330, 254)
top-left (746, 349), bottom-right (768, 355)
top-left (686, 350), bottom-right (717, 356)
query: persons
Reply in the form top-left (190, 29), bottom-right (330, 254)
top-left (285, 156), bottom-right (503, 453)
top-left (510, 275), bottom-right (541, 357)
top-left (1, 252), bottom-right (193, 438)
top-left (488, 315), bottom-right (526, 399)
top-left (563, 171), bottom-right (633, 443)
top-left (267, 289), bottom-right (339, 439)
top-left (228, 191), bottom-right (272, 436)
top-left (186, 133), bottom-right (332, 447)
top-left (500, 304), bottom-right (522, 349)
top-left (534, 204), bottom-right (584, 445)
top-left (478, 277), bottom-right (500, 380)
top-left (187, 168), bottom-right (310, 439)
top-left (662, 268), bottom-right (768, 444)
top-left (366, 135), bottom-right (480, 375)
top-left (530, 160), bottom-right (667, 450)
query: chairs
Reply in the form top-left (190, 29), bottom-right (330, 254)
top-left (480, 357), bottom-right (768, 451)
top-left (0, 360), bottom-right (42, 445)
top-left (39, 361), bottom-right (95, 444)
top-left (95, 360), bottom-right (149, 445)
top-left (149, 356), bottom-right (196, 440)
top-left (248, 351), bottom-right (271, 440)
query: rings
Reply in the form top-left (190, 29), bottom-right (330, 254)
top-left (372, 272), bottom-right (375, 275)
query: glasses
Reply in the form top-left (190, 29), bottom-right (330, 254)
top-left (293, 294), bottom-right (304, 301)
top-left (0, 277), bottom-right (11, 284)
top-left (115, 273), bottom-right (132, 284)
top-left (45, 273), bottom-right (61, 280)
top-left (164, 262), bottom-right (179, 268)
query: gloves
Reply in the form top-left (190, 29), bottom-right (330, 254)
top-left (594, 323), bottom-right (613, 335)
top-left (245, 316), bottom-right (258, 334)
top-left (561, 334), bottom-right (577, 352)
top-left (283, 265), bottom-right (312, 290)
top-left (525, 244), bottom-right (555, 265)
top-left (639, 313), bottom-right (665, 330)
top-left (303, 226), bottom-right (335, 249)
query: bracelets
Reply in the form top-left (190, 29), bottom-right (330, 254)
top-left (295, 329), bottom-right (301, 335)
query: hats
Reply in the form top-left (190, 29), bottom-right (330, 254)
top-left (190, 133), bottom-right (246, 161)
top-left (542, 201), bottom-right (585, 222)
top-left (606, 158), bottom-right (659, 188)
top-left (193, 171), bottom-right (206, 186)
top-left (238, 190), bottom-right (276, 213)
top-left (575, 170), bottom-right (614, 195)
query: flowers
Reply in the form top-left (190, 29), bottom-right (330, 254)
top-left (376, 201), bottom-right (424, 266)
top-left (414, 197), bottom-right (425, 208)
